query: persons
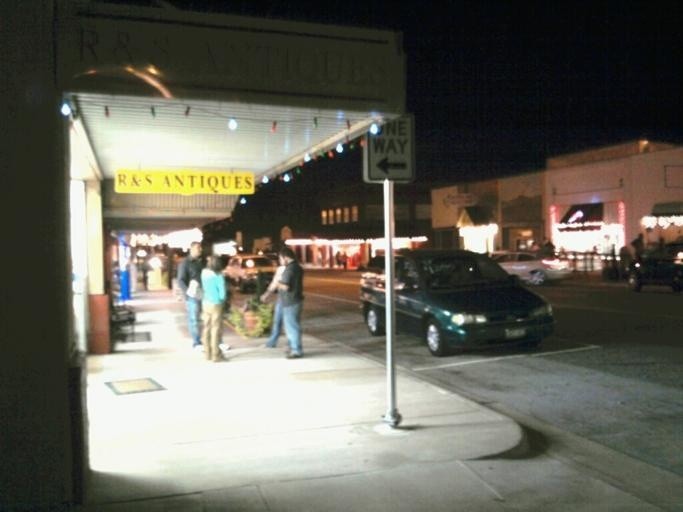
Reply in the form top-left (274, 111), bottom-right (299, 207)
top-left (336, 251), bottom-right (348, 268)
top-left (260, 248), bottom-right (303, 358)
top-left (201, 255), bottom-right (227, 362)
top-left (631, 233), bottom-right (644, 257)
top-left (177, 242), bottom-right (230, 350)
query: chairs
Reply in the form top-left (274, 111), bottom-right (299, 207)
top-left (104, 280), bottom-right (135, 344)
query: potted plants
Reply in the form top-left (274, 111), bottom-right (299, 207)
top-left (229, 297), bottom-right (275, 341)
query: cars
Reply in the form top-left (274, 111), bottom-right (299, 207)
top-left (222, 254), bottom-right (276, 293)
top-left (622, 242), bottom-right (683, 292)
top-left (360, 248), bottom-right (575, 357)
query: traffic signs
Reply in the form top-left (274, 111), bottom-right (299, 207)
top-left (363, 113), bottom-right (415, 184)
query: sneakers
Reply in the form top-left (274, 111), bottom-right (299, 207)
top-left (219, 343), bottom-right (231, 350)
top-left (194, 344), bottom-right (205, 352)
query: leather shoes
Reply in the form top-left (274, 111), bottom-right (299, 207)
top-left (286, 351), bottom-right (303, 359)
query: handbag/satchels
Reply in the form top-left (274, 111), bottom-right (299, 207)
top-left (186, 279), bottom-right (202, 300)
